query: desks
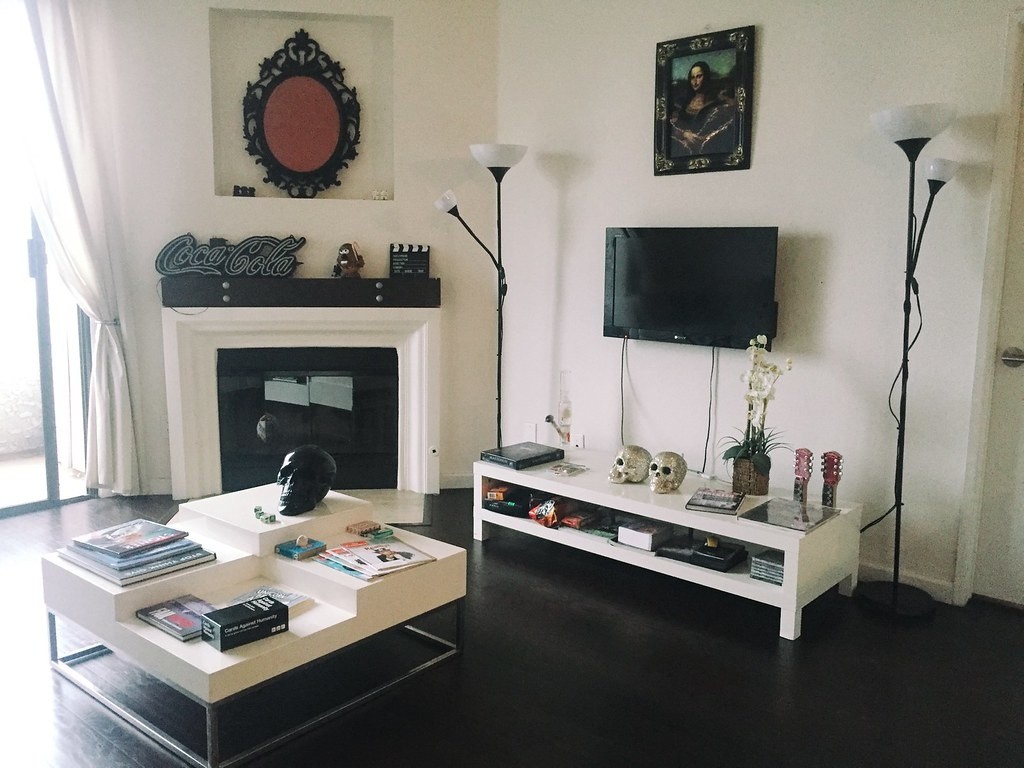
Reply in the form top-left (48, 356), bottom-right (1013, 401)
top-left (40, 481), bottom-right (468, 768)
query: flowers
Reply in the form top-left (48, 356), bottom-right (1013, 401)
top-left (714, 334), bottom-right (794, 478)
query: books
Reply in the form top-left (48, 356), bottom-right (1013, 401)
top-left (685, 486), bottom-right (747, 516)
top-left (135, 592), bottom-right (221, 642)
top-left (312, 535), bottom-right (438, 579)
top-left (54, 515), bottom-right (216, 588)
top-left (608, 518), bottom-right (674, 556)
top-left (226, 581), bottom-right (313, 621)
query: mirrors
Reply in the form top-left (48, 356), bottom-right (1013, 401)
top-left (242, 28), bottom-right (364, 200)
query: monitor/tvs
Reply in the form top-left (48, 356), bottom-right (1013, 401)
top-left (603, 227), bottom-right (779, 352)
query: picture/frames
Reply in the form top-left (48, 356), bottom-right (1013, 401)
top-left (653, 25), bottom-right (756, 175)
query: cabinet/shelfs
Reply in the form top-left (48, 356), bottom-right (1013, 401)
top-left (472, 441), bottom-right (862, 640)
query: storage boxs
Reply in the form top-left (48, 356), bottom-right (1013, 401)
top-left (618, 519), bottom-right (673, 550)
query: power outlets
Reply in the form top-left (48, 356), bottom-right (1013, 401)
top-left (569, 433), bottom-right (585, 448)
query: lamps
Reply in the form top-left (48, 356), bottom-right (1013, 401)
top-left (849, 102), bottom-right (961, 621)
top-left (434, 143), bottom-right (528, 443)
